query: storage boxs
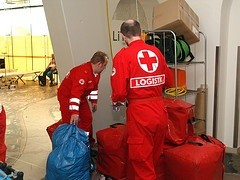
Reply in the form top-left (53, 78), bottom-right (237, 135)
top-left (152, 0), bottom-right (200, 46)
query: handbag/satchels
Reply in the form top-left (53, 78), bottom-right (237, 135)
top-left (162, 134), bottom-right (226, 180)
top-left (96, 123), bottom-right (128, 180)
top-left (163, 97), bottom-right (196, 145)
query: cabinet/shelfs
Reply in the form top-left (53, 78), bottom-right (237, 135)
top-left (141, 30), bottom-right (207, 136)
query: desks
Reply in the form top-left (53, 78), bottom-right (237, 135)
top-left (0, 71), bottom-right (45, 86)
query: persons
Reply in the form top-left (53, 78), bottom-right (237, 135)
top-left (41, 55), bottom-right (56, 87)
top-left (110, 19), bottom-right (173, 180)
top-left (57, 51), bottom-right (109, 157)
